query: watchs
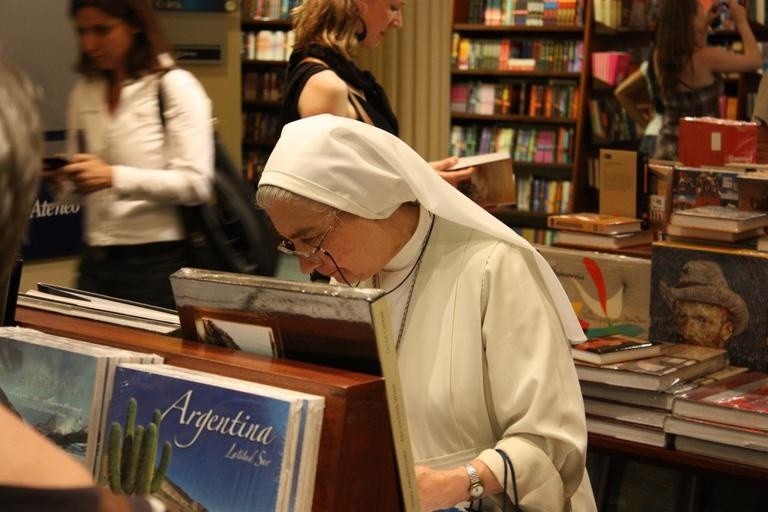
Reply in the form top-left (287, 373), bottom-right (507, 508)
top-left (461, 461), bottom-right (483, 500)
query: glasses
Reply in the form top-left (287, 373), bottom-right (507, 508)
top-left (277, 211), bottom-right (342, 262)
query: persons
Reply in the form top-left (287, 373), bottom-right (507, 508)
top-left (2, 55), bottom-right (106, 512)
top-left (274, 1), bottom-right (480, 285)
top-left (614, 0), bottom-right (762, 160)
top-left (40, 0), bottom-right (217, 311)
top-left (253, 112), bottom-right (599, 510)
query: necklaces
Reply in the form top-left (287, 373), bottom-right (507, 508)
top-left (395, 227), bottom-right (434, 353)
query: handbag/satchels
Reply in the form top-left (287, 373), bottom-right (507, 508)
top-left (178, 135), bottom-right (278, 275)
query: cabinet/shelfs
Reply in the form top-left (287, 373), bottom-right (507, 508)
top-left (160, 0), bottom-right (765, 511)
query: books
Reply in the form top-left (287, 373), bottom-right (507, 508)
top-left (14, 288), bottom-right (184, 340)
top-left (517, 175), bottom-right (572, 244)
top-left (702, 1), bottom-right (767, 120)
top-left (545, 118), bottom-right (768, 242)
top-left (449, 33), bottom-right (630, 86)
top-left (450, 80), bottom-right (607, 164)
top-left (166, 265), bottom-right (420, 512)
top-left (242, 0), bottom-right (304, 187)
top-left (24, 281), bottom-right (185, 323)
top-left (443, 150), bottom-right (517, 207)
top-left (538, 244), bottom-right (768, 467)
top-left (3, 324), bottom-right (325, 512)
top-left (467, 1), bottom-right (623, 29)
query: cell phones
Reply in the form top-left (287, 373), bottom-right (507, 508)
top-left (41, 157), bottom-right (70, 171)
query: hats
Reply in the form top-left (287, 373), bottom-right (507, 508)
top-left (660, 260), bottom-right (749, 336)
